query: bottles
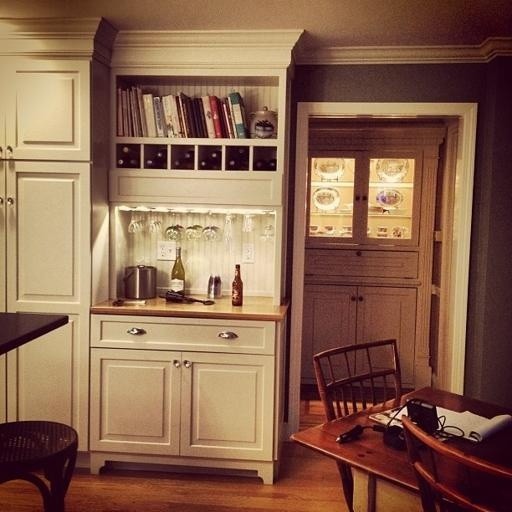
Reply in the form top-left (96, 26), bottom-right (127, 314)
top-left (247, 106), bottom-right (278, 139)
top-left (206, 275), bottom-right (222, 299)
top-left (170, 246), bottom-right (186, 299)
top-left (231, 265), bottom-right (244, 307)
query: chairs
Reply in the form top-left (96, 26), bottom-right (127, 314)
top-left (287, 339), bottom-right (512, 511)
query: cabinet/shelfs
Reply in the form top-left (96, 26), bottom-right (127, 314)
top-left (0, 16), bottom-right (89, 469)
top-left (105, 29), bottom-right (304, 305)
top-left (91, 348), bottom-right (276, 482)
top-left (90, 313), bottom-right (277, 351)
top-left (302, 126), bottom-right (450, 389)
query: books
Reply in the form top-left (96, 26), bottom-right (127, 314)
top-left (115, 83), bottom-right (251, 139)
top-left (388, 398), bottom-right (511, 444)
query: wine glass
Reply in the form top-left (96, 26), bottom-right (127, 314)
top-left (126, 207), bottom-right (275, 246)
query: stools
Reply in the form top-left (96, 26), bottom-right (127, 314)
top-left (0, 422), bottom-right (77, 511)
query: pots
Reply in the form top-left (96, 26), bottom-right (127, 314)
top-left (123, 265), bottom-right (158, 300)
